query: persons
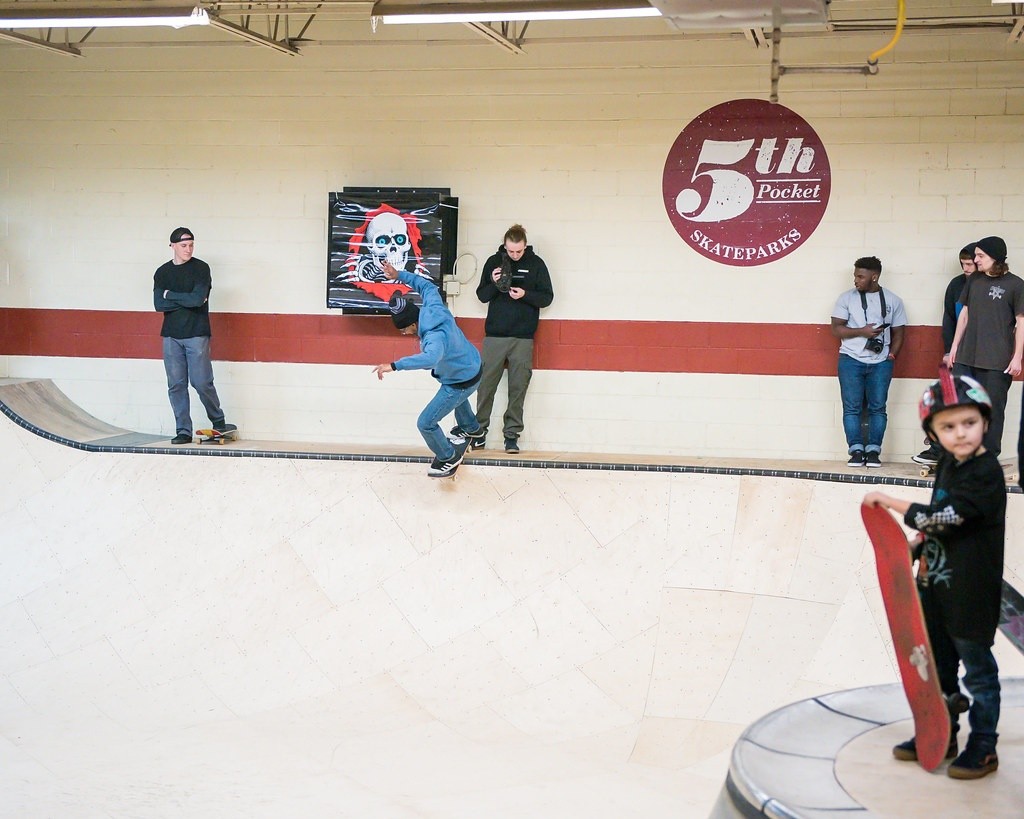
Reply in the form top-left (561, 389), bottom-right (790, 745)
top-left (831, 257), bottom-right (910, 468)
top-left (153, 228), bottom-right (226, 445)
top-left (863, 375), bottom-right (1007, 778)
top-left (915, 235), bottom-right (1024, 462)
top-left (470, 224), bottom-right (555, 454)
top-left (371, 261), bottom-right (485, 474)
top-left (941, 242), bottom-right (978, 372)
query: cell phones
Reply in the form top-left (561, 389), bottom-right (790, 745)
top-left (876, 323), bottom-right (890, 329)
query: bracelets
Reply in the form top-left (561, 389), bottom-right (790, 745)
top-left (889, 353), bottom-right (895, 359)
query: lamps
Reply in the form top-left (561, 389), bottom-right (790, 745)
top-left (0, 6), bottom-right (211, 27)
top-left (370, 0), bottom-right (662, 33)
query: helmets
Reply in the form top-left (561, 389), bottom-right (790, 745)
top-left (919, 377), bottom-right (993, 428)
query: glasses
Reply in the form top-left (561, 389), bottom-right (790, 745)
top-left (401, 328), bottom-right (406, 336)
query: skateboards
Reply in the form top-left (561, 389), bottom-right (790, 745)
top-left (196, 424), bottom-right (238, 445)
top-left (427, 437), bottom-right (473, 482)
top-left (910, 456), bottom-right (1014, 476)
top-left (860, 500), bottom-right (972, 774)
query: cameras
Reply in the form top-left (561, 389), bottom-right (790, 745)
top-left (497, 253), bottom-right (512, 293)
top-left (865, 338), bottom-right (883, 353)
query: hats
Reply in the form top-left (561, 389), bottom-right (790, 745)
top-left (976, 236), bottom-right (1007, 264)
top-left (169, 227), bottom-right (194, 246)
top-left (389, 297), bottom-right (420, 329)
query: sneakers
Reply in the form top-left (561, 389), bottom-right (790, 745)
top-left (504, 436), bottom-right (519, 453)
top-left (893, 732), bottom-right (959, 763)
top-left (948, 746), bottom-right (998, 780)
top-left (470, 438), bottom-right (486, 449)
top-left (912, 448), bottom-right (940, 465)
top-left (848, 452), bottom-right (866, 467)
top-left (428, 449), bottom-right (463, 474)
top-left (450, 425), bottom-right (484, 438)
top-left (171, 434), bottom-right (192, 444)
top-left (213, 419), bottom-right (226, 433)
top-left (866, 453), bottom-right (881, 467)
